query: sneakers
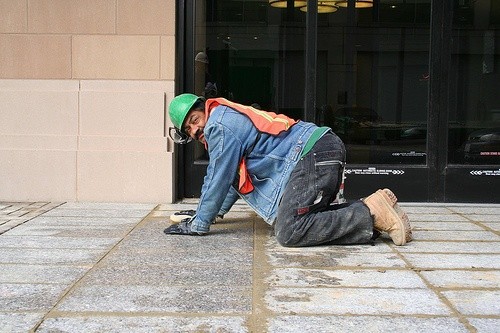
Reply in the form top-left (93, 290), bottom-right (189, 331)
top-left (363, 188), bottom-right (413, 245)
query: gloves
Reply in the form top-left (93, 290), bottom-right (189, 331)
top-left (175, 209), bottom-right (196, 218)
top-left (164, 220), bottom-right (201, 236)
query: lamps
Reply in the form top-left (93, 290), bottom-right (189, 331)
top-left (269, 0), bottom-right (375, 14)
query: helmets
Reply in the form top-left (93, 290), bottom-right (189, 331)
top-left (169, 94), bottom-right (204, 132)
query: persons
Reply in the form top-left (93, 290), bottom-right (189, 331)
top-left (163, 94), bottom-right (412, 247)
